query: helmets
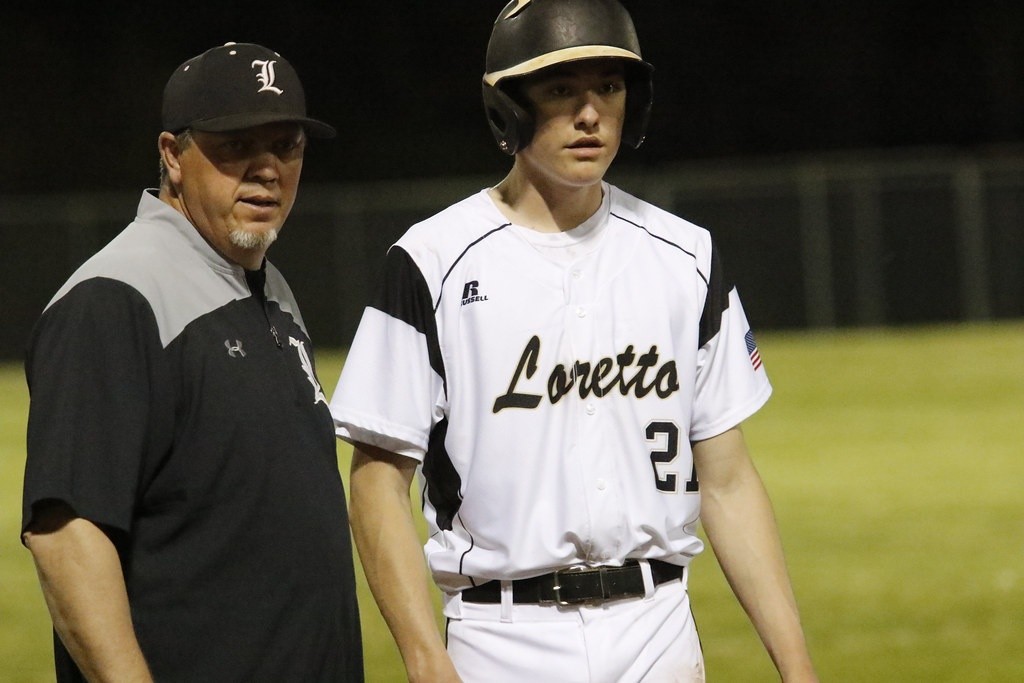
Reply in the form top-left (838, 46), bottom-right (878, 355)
top-left (482, 0), bottom-right (655, 155)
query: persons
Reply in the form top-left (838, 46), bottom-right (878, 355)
top-left (22, 44), bottom-right (366, 683)
top-left (331, 2), bottom-right (821, 683)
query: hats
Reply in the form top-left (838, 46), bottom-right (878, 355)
top-left (161, 42), bottom-right (338, 140)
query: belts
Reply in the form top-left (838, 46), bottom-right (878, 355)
top-left (462, 559), bottom-right (683, 606)
